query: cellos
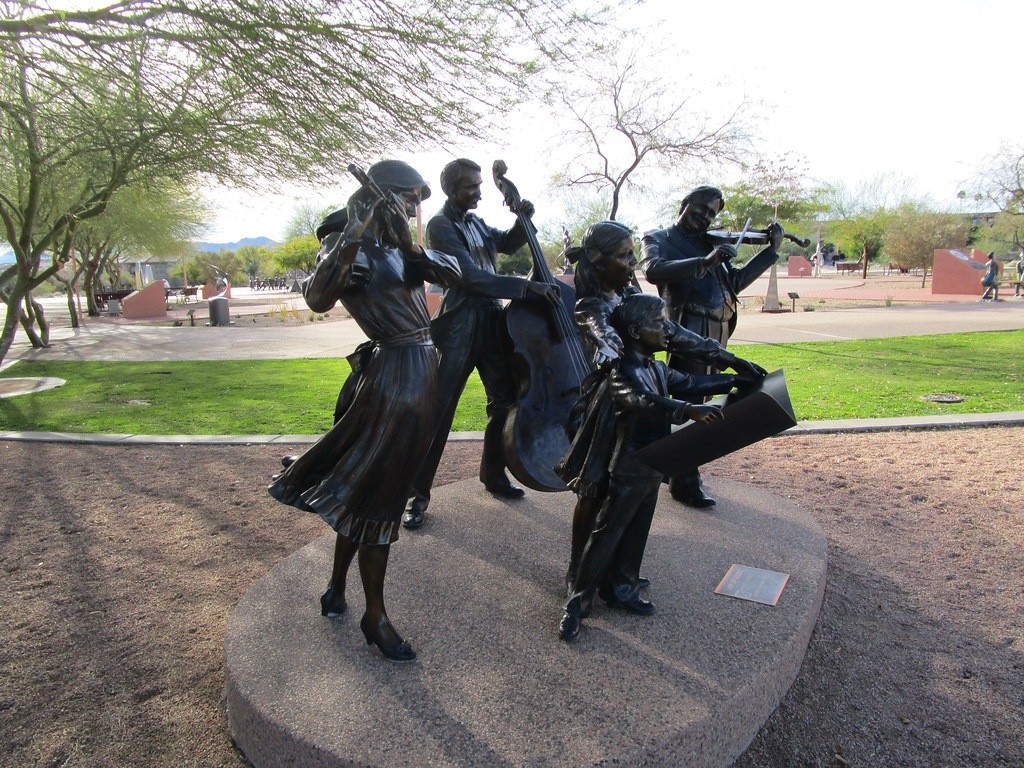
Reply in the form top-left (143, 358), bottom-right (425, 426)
top-left (490, 158), bottom-right (599, 492)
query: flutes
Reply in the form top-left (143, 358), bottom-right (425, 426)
top-left (347, 164), bottom-right (398, 215)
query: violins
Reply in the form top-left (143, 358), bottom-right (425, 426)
top-left (705, 228), bottom-right (811, 248)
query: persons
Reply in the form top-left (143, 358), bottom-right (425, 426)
top-left (1015, 252), bottom-right (1024, 296)
top-left (642, 187), bottom-right (784, 509)
top-left (247, 277), bottom-right (287, 291)
top-left (402, 159), bottom-right (562, 531)
top-left (557, 221), bottom-right (767, 641)
top-left (267, 161), bottom-right (462, 663)
top-left (981, 252), bottom-right (1002, 302)
top-left (162, 279), bottom-right (171, 288)
